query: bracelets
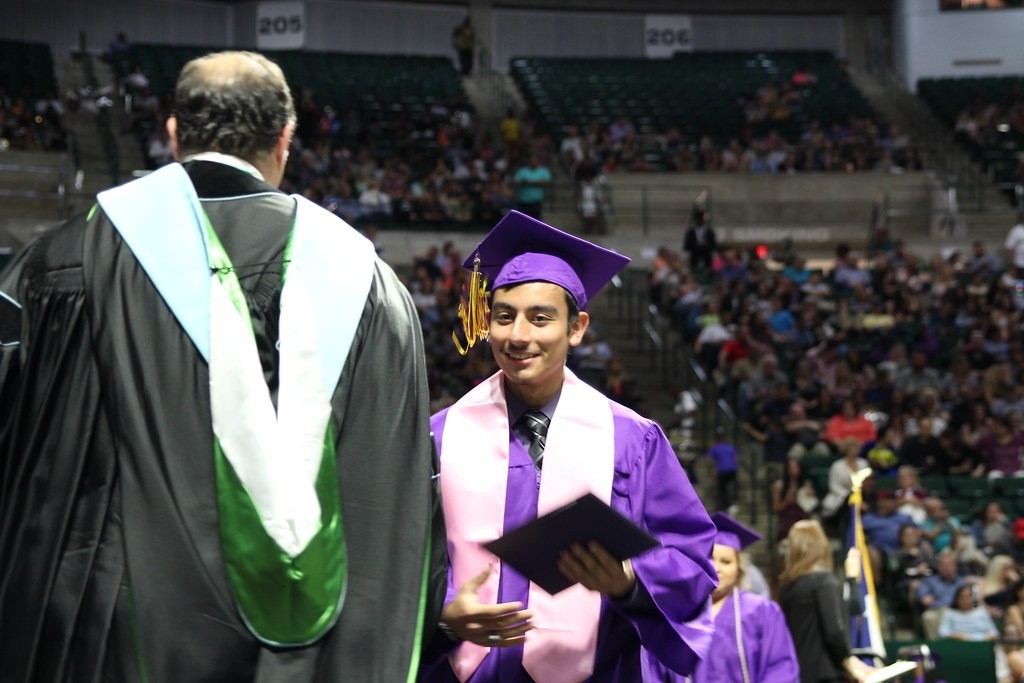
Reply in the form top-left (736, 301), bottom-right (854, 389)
top-left (438, 620), bottom-right (459, 641)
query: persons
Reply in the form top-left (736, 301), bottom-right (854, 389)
top-left (954, 83), bottom-right (1024, 206)
top-left (639, 510), bottom-right (802, 683)
top-left (0, 88), bottom-right (67, 152)
top-left (396, 242), bottom-right (639, 415)
top-left (0, 50), bottom-right (448, 683)
top-left (430, 208), bottom-right (719, 683)
top-left (71, 32), bottom-right (922, 232)
top-left (649, 209), bottom-right (1024, 683)
top-left (452, 17), bottom-right (476, 75)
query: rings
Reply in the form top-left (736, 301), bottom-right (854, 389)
top-left (487, 633), bottom-right (502, 642)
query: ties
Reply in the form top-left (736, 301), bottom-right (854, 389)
top-left (519, 412), bottom-right (550, 470)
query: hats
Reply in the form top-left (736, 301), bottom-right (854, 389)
top-left (708, 512), bottom-right (764, 552)
top-left (452, 209), bottom-right (631, 357)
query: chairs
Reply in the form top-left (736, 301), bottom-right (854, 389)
top-left (113, 38), bottom-right (864, 137)
